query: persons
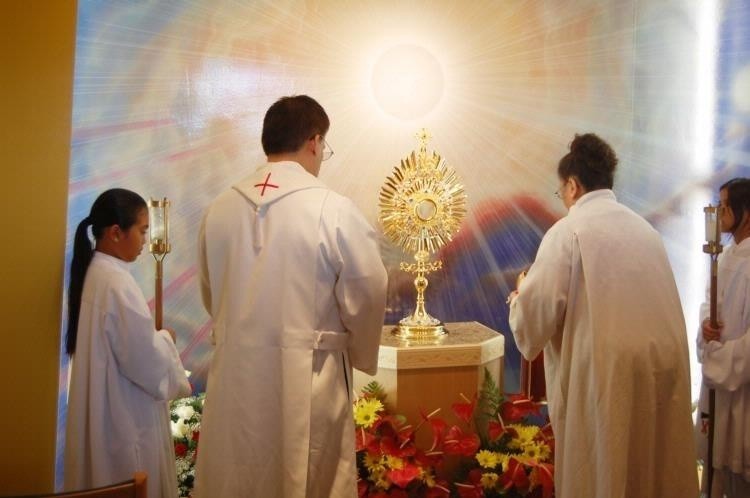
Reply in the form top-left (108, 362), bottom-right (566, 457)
top-left (62, 186), bottom-right (194, 497)
top-left (694, 176), bottom-right (748, 497)
top-left (503, 131), bottom-right (703, 498)
top-left (196, 94), bottom-right (391, 497)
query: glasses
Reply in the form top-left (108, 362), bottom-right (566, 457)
top-left (555, 177), bottom-right (578, 199)
top-left (309, 134), bottom-right (333, 160)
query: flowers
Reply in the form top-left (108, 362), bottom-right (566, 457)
top-left (352, 382), bottom-right (556, 496)
top-left (167, 393), bottom-right (206, 496)
